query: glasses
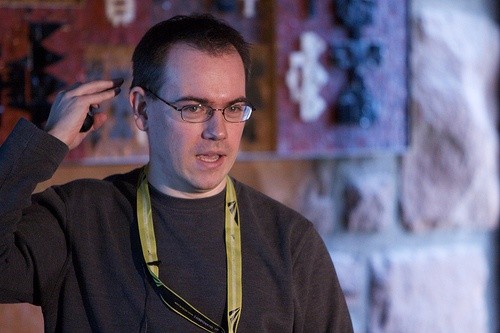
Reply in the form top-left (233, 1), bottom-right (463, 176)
top-left (138, 84), bottom-right (256, 123)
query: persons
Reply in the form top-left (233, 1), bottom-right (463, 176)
top-left (0, 14), bottom-right (354, 333)
